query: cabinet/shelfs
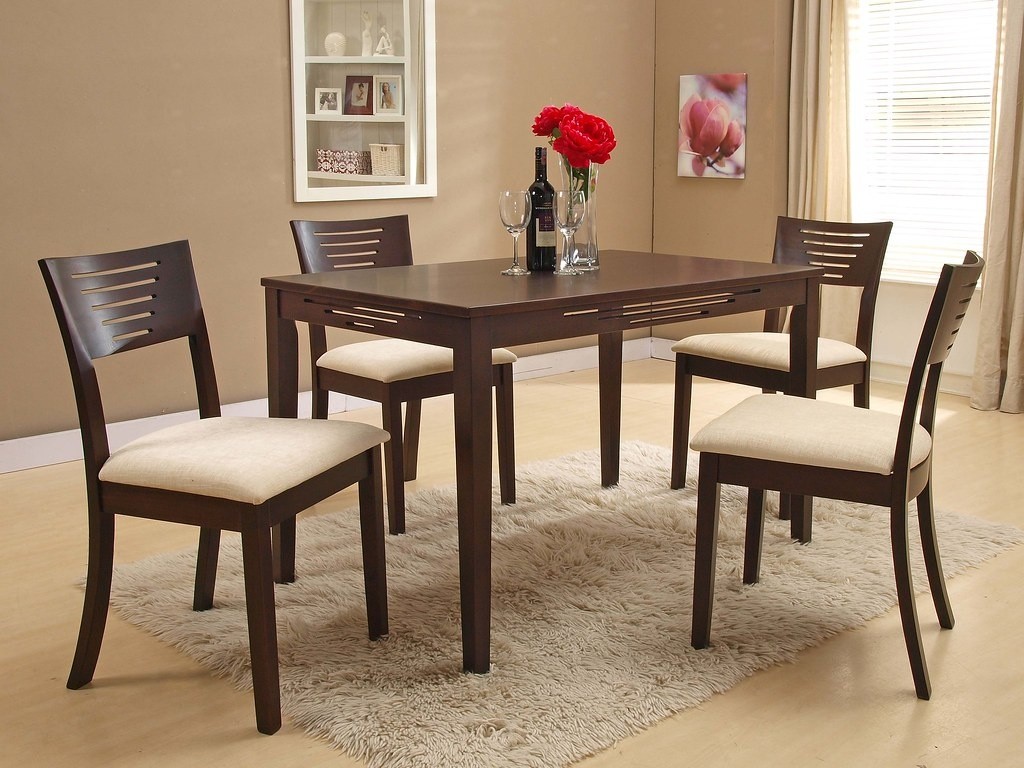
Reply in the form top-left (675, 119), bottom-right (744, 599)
top-left (302, 1), bottom-right (423, 187)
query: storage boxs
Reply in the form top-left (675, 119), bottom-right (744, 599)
top-left (316, 148), bottom-right (372, 175)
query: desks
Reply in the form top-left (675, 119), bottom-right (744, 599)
top-left (261, 249), bottom-right (826, 674)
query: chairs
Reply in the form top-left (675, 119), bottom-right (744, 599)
top-left (689, 249), bottom-right (985, 700)
top-left (37, 240), bottom-right (392, 735)
top-left (671, 215), bottom-right (893, 520)
top-left (290, 213), bottom-right (518, 535)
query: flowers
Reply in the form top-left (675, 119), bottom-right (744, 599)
top-left (531, 103), bottom-right (616, 216)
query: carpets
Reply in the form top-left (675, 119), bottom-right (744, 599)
top-left (70, 436), bottom-right (1024, 767)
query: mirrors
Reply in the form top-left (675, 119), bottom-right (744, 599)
top-left (289, 0), bottom-right (437, 202)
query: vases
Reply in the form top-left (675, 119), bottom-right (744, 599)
top-left (557, 153), bottom-right (600, 271)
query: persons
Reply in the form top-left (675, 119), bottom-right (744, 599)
top-left (375, 26), bottom-right (393, 54)
top-left (355, 83), bottom-right (366, 104)
top-left (380, 82), bottom-right (396, 109)
top-left (360, 8), bottom-right (372, 55)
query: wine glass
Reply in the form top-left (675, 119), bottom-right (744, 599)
top-left (499, 189), bottom-right (533, 278)
top-left (554, 191), bottom-right (586, 274)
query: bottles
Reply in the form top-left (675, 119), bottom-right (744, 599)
top-left (526, 146), bottom-right (557, 271)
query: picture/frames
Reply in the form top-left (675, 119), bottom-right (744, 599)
top-left (373, 75), bottom-right (404, 115)
top-left (314, 88), bottom-right (343, 115)
top-left (344, 76), bottom-right (373, 115)
